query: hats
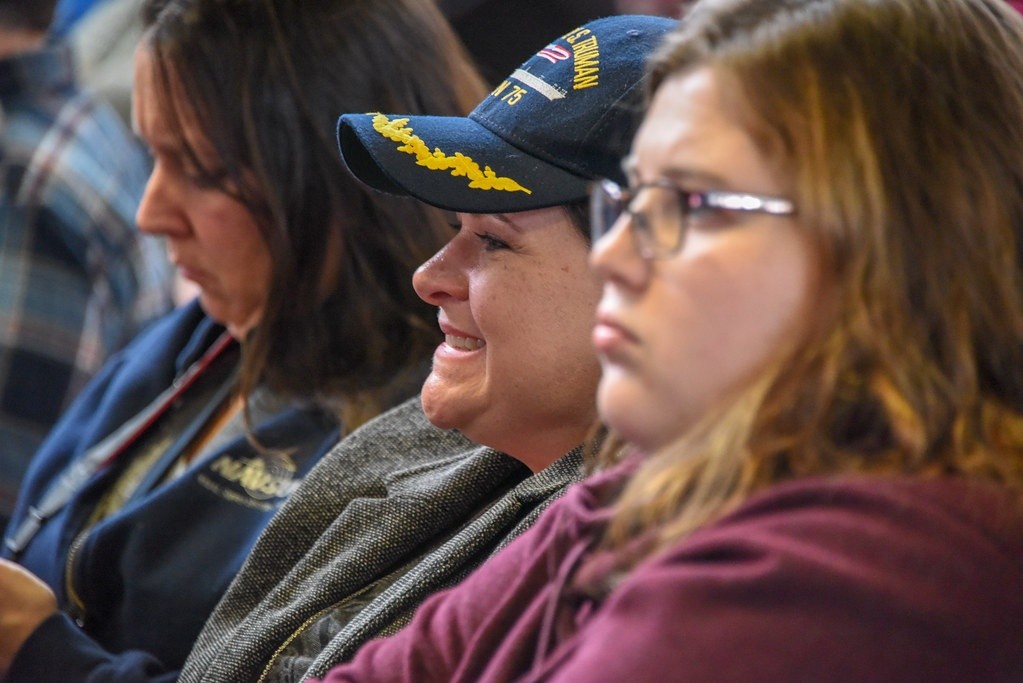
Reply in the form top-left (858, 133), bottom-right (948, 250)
top-left (335, 16), bottom-right (692, 238)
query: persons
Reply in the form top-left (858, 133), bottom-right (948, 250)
top-left (178, 15), bottom-right (688, 683)
top-left (0, 0), bottom-right (492, 683)
top-left (299, 1), bottom-right (1023, 683)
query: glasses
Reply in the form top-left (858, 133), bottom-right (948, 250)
top-left (588, 181), bottom-right (800, 260)
